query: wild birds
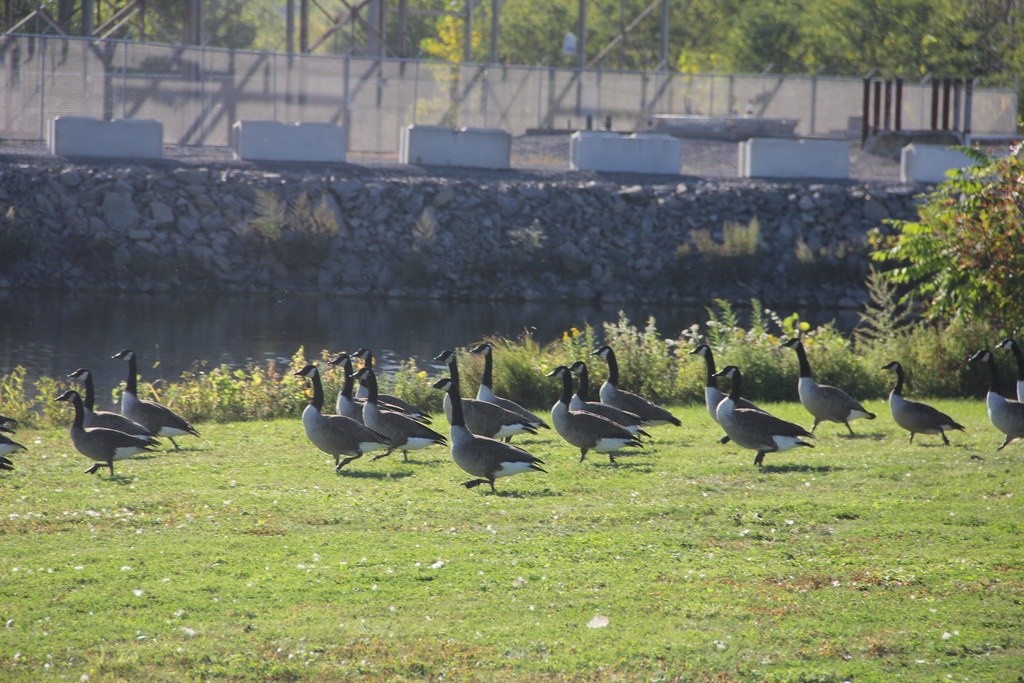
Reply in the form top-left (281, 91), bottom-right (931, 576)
top-left (0, 338), bottom-right (1023, 495)
